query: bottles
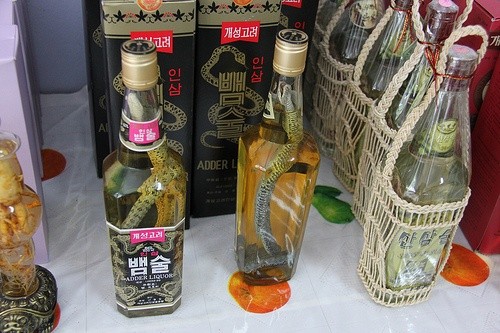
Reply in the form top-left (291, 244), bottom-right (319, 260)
top-left (306, 0), bottom-right (478, 307)
top-left (229, 29), bottom-right (320, 313)
top-left (102, 39), bottom-right (184, 319)
top-left (0, 131), bottom-right (43, 296)
